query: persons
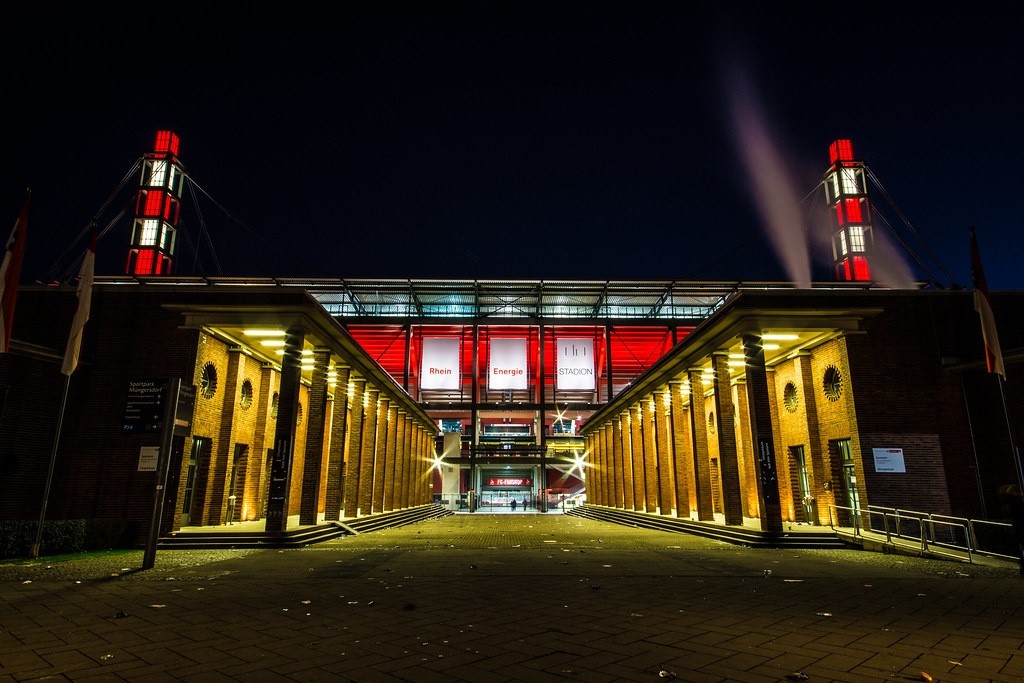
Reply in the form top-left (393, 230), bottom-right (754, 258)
top-left (510, 500), bottom-right (517, 511)
top-left (522, 499), bottom-right (528, 511)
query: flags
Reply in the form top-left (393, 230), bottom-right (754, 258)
top-left (970, 236), bottom-right (1007, 380)
top-left (0, 198), bottom-right (32, 353)
top-left (61, 231), bottom-right (97, 377)
